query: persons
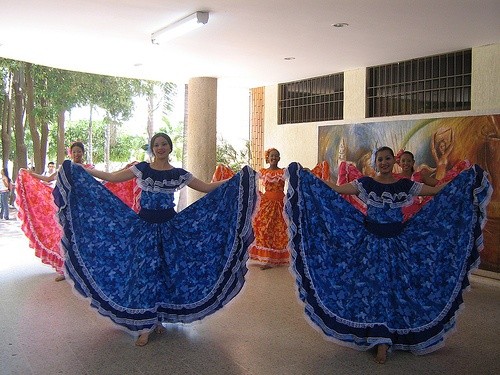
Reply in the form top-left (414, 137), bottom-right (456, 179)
top-left (0, 131), bottom-right (493, 363)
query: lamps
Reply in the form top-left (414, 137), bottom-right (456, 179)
top-left (151, 12), bottom-right (209, 45)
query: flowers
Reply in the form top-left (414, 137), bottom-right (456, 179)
top-left (396, 149), bottom-right (404, 159)
